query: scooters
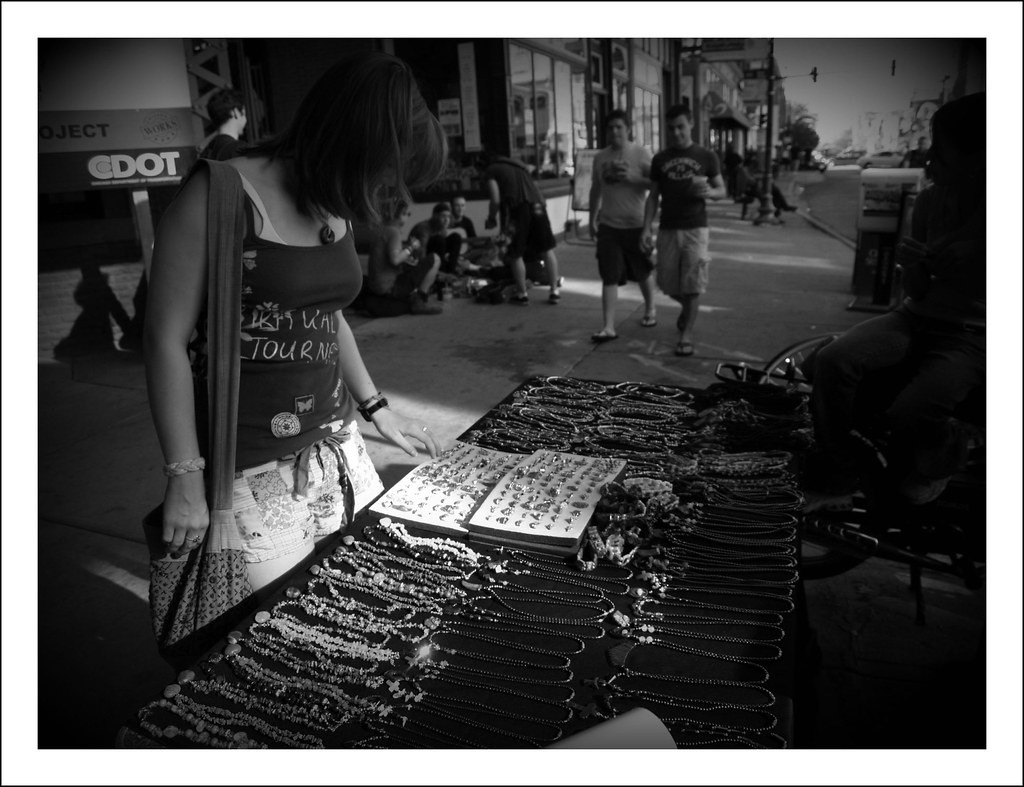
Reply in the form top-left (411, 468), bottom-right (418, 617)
top-left (712, 361), bottom-right (986, 580)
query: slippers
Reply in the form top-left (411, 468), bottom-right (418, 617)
top-left (641, 316), bottom-right (657, 327)
top-left (677, 316), bottom-right (686, 331)
top-left (591, 331), bottom-right (617, 340)
top-left (675, 342), bottom-right (694, 356)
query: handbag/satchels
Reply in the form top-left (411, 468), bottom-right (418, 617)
top-left (143, 500), bottom-right (257, 667)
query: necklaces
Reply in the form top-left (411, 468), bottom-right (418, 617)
top-left (314, 208), bottom-right (335, 244)
top-left (117, 376), bottom-right (803, 749)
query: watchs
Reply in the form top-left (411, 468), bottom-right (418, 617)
top-left (361, 398), bottom-right (388, 422)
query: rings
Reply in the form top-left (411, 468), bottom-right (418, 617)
top-left (422, 427), bottom-right (427, 431)
top-left (186, 536), bottom-right (201, 544)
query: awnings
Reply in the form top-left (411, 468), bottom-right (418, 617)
top-left (704, 91), bottom-right (751, 129)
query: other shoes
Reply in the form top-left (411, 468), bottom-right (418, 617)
top-left (415, 298), bottom-right (446, 313)
top-left (509, 295), bottom-right (529, 305)
top-left (548, 294), bottom-right (561, 304)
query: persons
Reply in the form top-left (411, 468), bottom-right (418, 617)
top-left (639, 104), bottom-right (726, 356)
top-left (724, 141), bottom-right (800, 223)
top-left (200, 89), bottom-right (247, 160)
top-left (350, 148), bottom-right (561, 316)
top-left (899, 136), bottom-right (929, 168)
top-left (590, 110), bottom-right (656, 340)
top-left (811, 94), bottom-right (987, 526)
top-left (144, 57), bottom-right (449, 603)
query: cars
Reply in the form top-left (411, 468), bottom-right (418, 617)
top-left (856, 150), bottom-right (909, 169)
top-left (806, 149), bottom-right (829, 173)
top-left (833, 148), bottom-right (867, 166)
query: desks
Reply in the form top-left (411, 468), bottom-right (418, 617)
top-left (119, 377), bottom-right (820, 749)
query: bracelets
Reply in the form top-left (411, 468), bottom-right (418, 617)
top-left (903, 262), bottom-right (917, 268)
top-left (162, 457), bottom-right (205, 476)
top-left (357, 393), bottom-right (383, 412)
